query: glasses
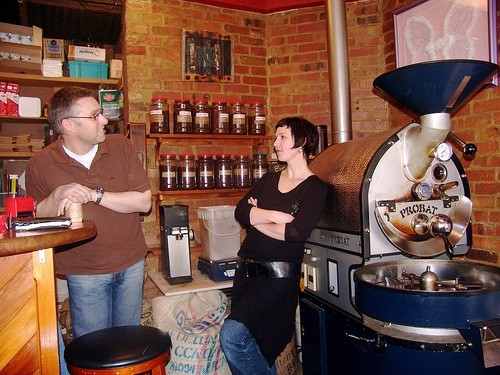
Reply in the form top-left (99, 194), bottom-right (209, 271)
top-left (60, 109), bottom-right (103, 124)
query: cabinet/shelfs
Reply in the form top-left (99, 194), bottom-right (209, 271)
top-left (147, 134), bottom-right (273, 194)
top-left (0, 0), bottom-right (127, 157)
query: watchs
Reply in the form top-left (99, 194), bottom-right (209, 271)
top-left (95, 187), bottom-right (105, 205)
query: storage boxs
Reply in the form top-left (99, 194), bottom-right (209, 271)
top-left (0, 22), bottom-right (108, 80)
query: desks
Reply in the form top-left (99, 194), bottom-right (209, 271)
top-left (0, 217), bottom-right (98, 375)
top-left (147, 267), bottom-right (235, 297)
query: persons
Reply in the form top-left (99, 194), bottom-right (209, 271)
top-left (25, 85), bottom-right (151, 340)
top-left (220, 116), bottom-right (329, 375)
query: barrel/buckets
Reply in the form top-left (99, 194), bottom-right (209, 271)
top-left (197, 205), bottom-right (243, 261)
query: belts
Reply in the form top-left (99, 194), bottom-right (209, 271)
top-left (236, 256), bottom-right (302, 280)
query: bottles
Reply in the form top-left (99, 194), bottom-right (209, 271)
top-left (158, 153), bottom-right (269, 189)
top-left (149, 97), bottom-right (266, 135)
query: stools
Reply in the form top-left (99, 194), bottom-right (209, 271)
top-left (63, 326), bottom-right (173, 375)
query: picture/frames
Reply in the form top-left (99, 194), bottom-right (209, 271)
top-left (181, 29), bottom-right (235, 83)
